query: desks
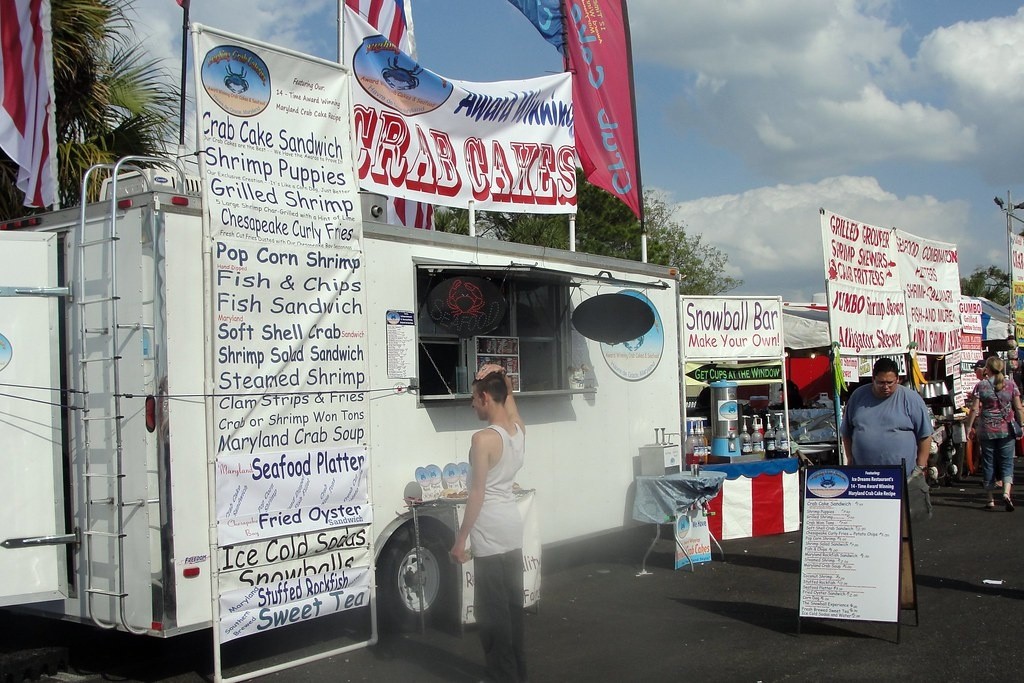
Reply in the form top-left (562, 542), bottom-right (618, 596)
top-left (687, 457), bottom-right (800, 542)
top-left (633, 471), bottom-right (728, 576)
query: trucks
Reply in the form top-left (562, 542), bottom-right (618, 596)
top-left (0, 143), bottom-right (689, 649)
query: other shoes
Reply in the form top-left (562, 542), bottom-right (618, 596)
top-left (1002, 493), bottom-right (1015, 512)
top-left (986, 499), bottom-right (995, 507)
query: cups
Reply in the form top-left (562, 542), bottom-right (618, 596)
top-left (691, 464), bottom-right (699, 477)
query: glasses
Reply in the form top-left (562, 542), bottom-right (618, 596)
top-left (874, 377), bottom-right (897, 387)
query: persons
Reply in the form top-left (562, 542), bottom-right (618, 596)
top-left (1005, 335), bottom-right (1024, 360)
top-left (773, 380), bottom-right (801, 409)
top-left (451, 364), bottom-right (529, 683)
top-left (838, 359), bottom-right (934, 524)
top-left (965, 356), bottom-right (1024, 511)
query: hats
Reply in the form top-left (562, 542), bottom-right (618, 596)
top-left (970, 359), bottom-right (986, 368)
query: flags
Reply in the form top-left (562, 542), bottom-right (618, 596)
top-left (342, 0), bottom-right (435, 233)
top-left (0, 0), bottom-right (62, 209)
top-left (509, 0), bottom-right (641, 219)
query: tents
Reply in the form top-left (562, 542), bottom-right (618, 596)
top-left (782, 293), bottom-right (1015, 465)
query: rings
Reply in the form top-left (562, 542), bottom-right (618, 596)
top-left (482, 367), bottom-right (486, 371)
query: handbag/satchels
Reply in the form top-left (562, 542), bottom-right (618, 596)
top-left (1009, 418), bottom-right (1023, 438)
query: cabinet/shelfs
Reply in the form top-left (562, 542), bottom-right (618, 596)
top-left (474, 337), bottom-right (520, 394)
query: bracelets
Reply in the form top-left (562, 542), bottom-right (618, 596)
top-left (919, 465), bottom-right (928, 471)
